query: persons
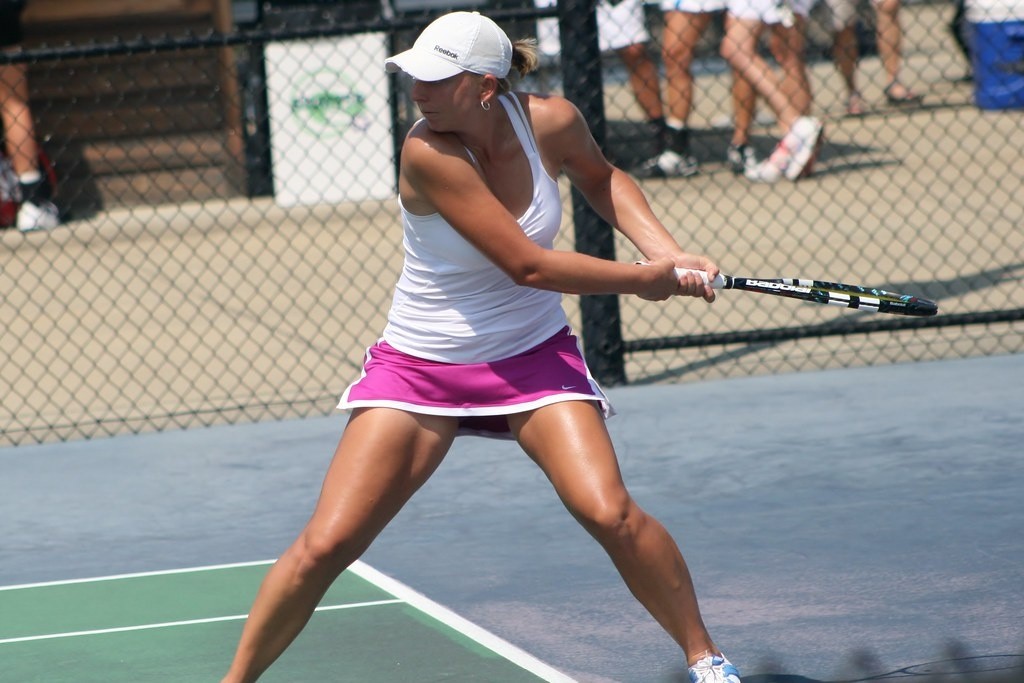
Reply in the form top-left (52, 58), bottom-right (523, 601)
top-left (221, 11), bottom-right (741, 683)
top-left (0, 0), bottom-right (61, 232)
top-left (537, 0), bottom-right (919, 183)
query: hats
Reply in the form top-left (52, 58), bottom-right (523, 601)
top-left (385, 9), bottom-right (515, 83)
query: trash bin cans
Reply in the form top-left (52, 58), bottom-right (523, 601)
top-left (965, 20), bottom-right (1024, 111)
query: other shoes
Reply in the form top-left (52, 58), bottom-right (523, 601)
top-left (882, 79), bottom-right (925, 109)
top-left (657, 116), bottom-right (692, 176)
top-left (632, 117), bottom-right (664, 178)
top-left (724, 141), bottom-right (745, 176)
top-left (843, 89), bottom-right (872, 117)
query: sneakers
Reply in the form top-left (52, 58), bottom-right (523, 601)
top-left (745, 116), bottom-right (823, 182)
top-left (687, 650), bottom-right (741, 683)
top-left (16, 197), bottom-right (58, 232)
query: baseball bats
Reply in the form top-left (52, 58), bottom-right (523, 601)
top-left (632, 262), bottom-right (941, 314)
